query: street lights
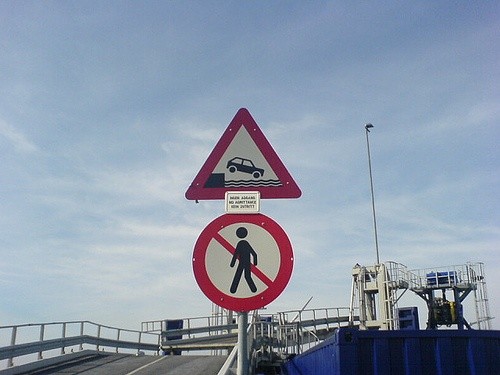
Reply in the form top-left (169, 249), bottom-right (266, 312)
top-left (364, 123), bottom-right (381, 266)
